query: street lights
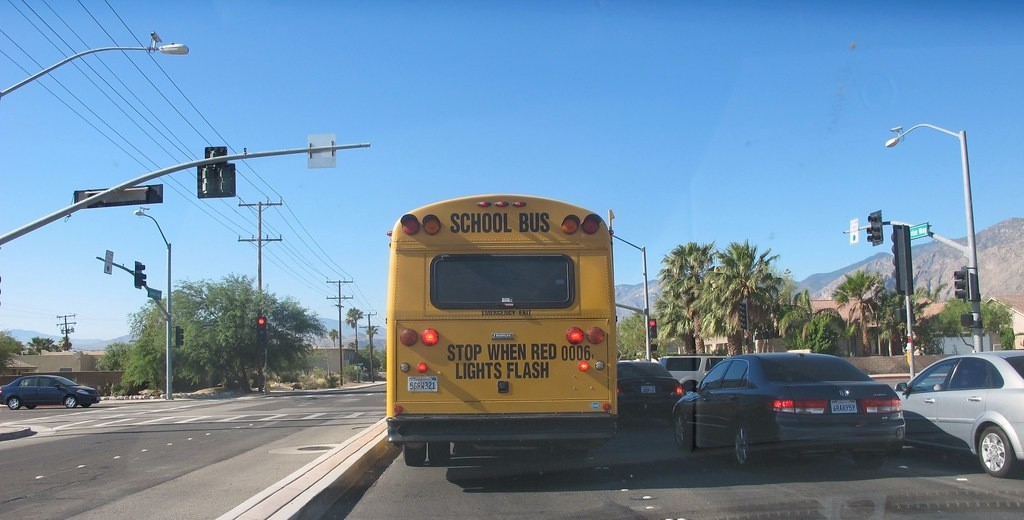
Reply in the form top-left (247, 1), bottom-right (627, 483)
top-left (885, 123), bottom-right (984, 353)
top-left (135, 210), bottom-right (172, 400)
top-left (0, 45), bottom-right (190, 96)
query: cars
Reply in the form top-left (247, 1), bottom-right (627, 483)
top-left (891, 351), bottom-right (1023, 478)
top-left (661, 354), bottom-right (729, 392)
top-left (672, 351), bottom-right (904, 467)
top-left (0, 374), bottom-right (101, 409)
top-left (614, 361), bottom-right (684, 438)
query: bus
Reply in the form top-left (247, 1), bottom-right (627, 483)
top-left (389, 193), bottom-right (620, 470)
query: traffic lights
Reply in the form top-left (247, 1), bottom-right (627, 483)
top-left (890, 226), bottom-right (914, 297)
top-left (648, 319), bottom-right (656, 337)
top-left (651, 344), bottom-right (657, 351)
top-left (255, 317), bottom-right (266, 348)
top-left (954, 266), bottom-right (968, 304)
top-left (960, 313), bottom-right (975, 329)
top-left (135, 261), bottom-right (146, 290)
top-left (738, 300), bottom-right (748, 331)
top-left (867, 210), bottom-right (884, 246)
top-left (174, 326), bottom-right (184, 349)
